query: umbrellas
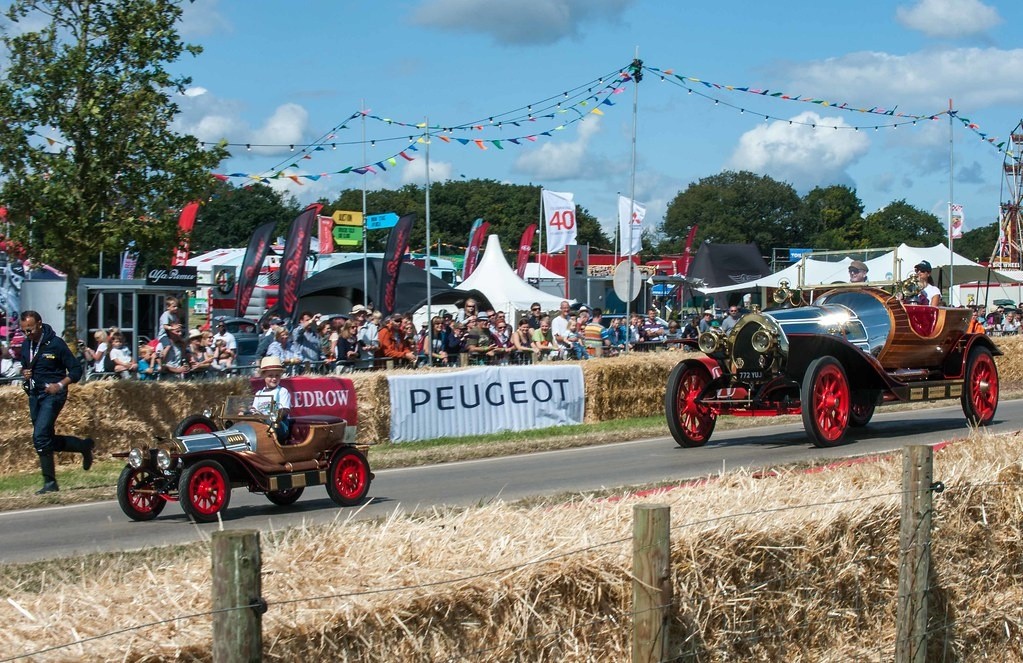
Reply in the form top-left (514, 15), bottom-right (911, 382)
top-left (450, 235), bottom-right (576, 318)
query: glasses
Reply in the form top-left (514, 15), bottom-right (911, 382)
top-left (349, 326), bottom-right (358, 329)
top-left (490, 314), bottom-right (494, 317)
top-left (915, 268), bottom-right (925, 273)
top-left (532, 308), bottom-right (540, 311)
top-left (468, 306), bottom-right (474, 308)
top-left (21, 323), bottom-right (38, 333)
top-left (499, 326), bottom-right (506, 329)
top-left (849, 269), bottom-right (866, 273)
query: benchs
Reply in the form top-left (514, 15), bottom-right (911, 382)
top-left (286, 418), bottom-right (328, 445)
top-left (903, 304), bottom-right (939, 337)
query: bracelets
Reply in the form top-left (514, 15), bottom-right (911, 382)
top-left (502, 348), bottom-right (505, 352)
top-left (528, 348), bottom-right (532, 352)
top-left (436, 354), bottom-right (441, 359)
top-left (484, 346), bottom-right (489, 352)
top-left (57, 382), bottom-right (63, 391)
top-left (291, 359), bottom-right (293, 364)
top-left (551, 347), bottom-right (555, 351)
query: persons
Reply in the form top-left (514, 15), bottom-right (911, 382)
top-left (421, 298), bottom-right (680, 365)
top-left (0, 334), bottom-right (28, 379)
top-left (77, 297), bottom-right (238, 381)
top-left (682, 306), bottom-right (739, 352)
top-left (910, 260), bottom-right (941, 309)
top-left (255, 299), bottom-right (420, 378)
top-left (848, 260), bottom-right (868, 283)
top-left (19, 310), bottom-right (95, 496)
top-left (967, 303), bottom-right (1023, 334)
top-left (250, 357), bottom-right (291, 441)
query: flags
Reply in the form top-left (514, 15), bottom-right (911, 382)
top-left (543, 190), bottom-right (578, 254)
top-left (619, 196), bottom-right (647, 258)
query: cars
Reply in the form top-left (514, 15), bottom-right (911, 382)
top-left (214, 315), bottom-right (265, 356)
top-left (111, 394), bottom-right (375, 527)
top-left (663, 264), bottom-right (1004, 449)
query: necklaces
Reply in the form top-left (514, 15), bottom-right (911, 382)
top-left (30, 336), bottom-right (42, 362)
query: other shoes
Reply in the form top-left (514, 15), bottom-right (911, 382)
top-left (35, 481), bottom-right (59, 496)
top-left (82, 438), bottom-right (94, 471)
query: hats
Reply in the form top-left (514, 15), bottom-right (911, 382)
top-left (258, 356), bottom-right (285, 375)
top-left (269, 317), bottom-right (285, 325)
top-left (390, 313), bottom-right (404, 319)
top-left (476, 311), bottom-right (490, 320)
top-left (187, 330), bottom-right (204, 339)
top-left (703, 310), bottom-right (714, 317)
top-left (914, 259), bottom-right (932, 272)
top-left (578, 306), bottom-right (589, 314)
top-left (848, 260), bottom-right (869, 272)
top-left (348, 305), bottom-right (372, 315)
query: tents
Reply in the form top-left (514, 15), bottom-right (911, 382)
top-left (256, 255), bottom-right (494, 324)
top-left (690, 242), bottom-right (1023, 296)
